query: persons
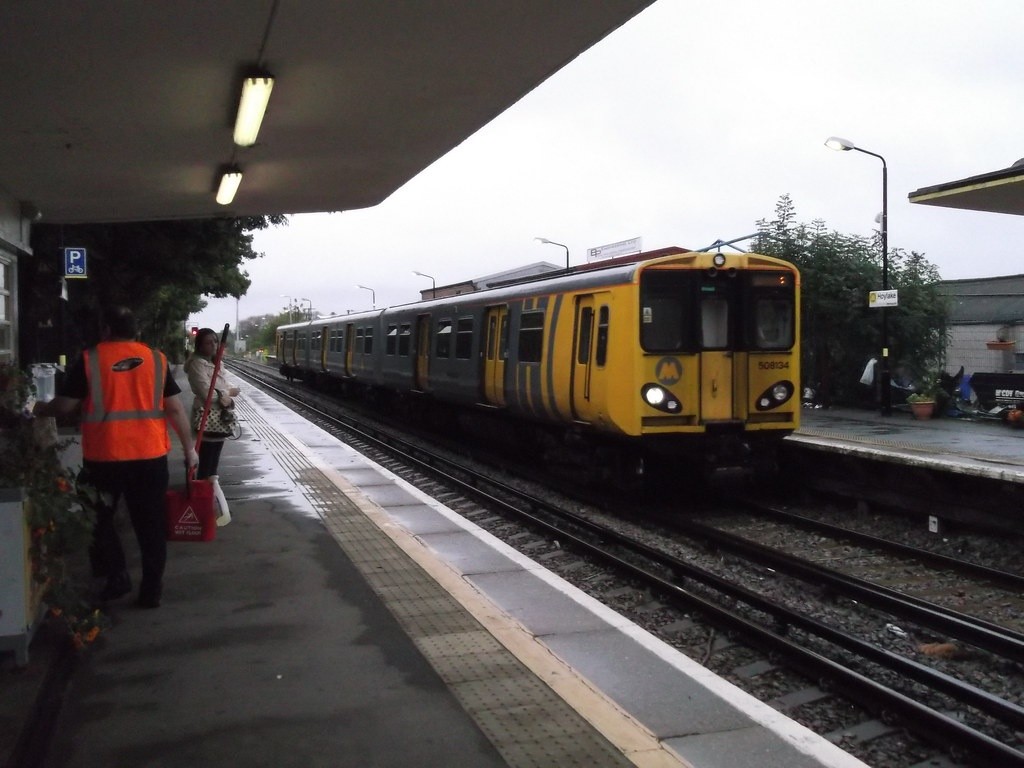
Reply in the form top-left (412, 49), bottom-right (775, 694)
top-left (34, 305), bottom-right (199, 608)
top-left (804, 382), bottom-right (832, 407)
top-left (184, 329), bottom-right (238, 477)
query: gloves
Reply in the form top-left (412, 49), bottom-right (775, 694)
top-left (183, 448), bottom-right (199, 474)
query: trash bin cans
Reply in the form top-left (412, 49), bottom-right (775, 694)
top-left (30, 362), bottom-right (57, 403)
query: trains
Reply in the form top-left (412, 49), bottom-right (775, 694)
top-left (275, 231), bottom-right (802, 446)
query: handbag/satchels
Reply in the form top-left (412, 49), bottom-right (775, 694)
top-left (193, 389), bottom-right (239, 438)
top-left (165, 463), bottom-right (217, 544)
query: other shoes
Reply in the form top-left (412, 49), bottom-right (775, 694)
top-left (138, 579), bottom-right (160, 608)
top-left (95, 589), bottom-right (126, 610)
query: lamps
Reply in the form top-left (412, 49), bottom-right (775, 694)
top-left (215, 169), bottom-right (243, 206)
top-left (234, 68), bottom-right (276, 147)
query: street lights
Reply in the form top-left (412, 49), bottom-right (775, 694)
top-left (532, 237), bottom-right (569, 277)
top-left (824, 135), bottom-right (891, 413)
top-left (409, 268), bottom-right (435, 300)
top-left (280, 295), bottom-right (313, 321)
top-left (356, 282), bottom-right (376, 312)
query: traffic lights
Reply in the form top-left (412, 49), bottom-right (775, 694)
top-left (191, 326), bottom-right (198, 337)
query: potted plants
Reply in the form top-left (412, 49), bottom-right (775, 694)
top-left (907, 393), bottom-right (936, 422)
top-left (987, 334), bottom-right (1015, 350)
